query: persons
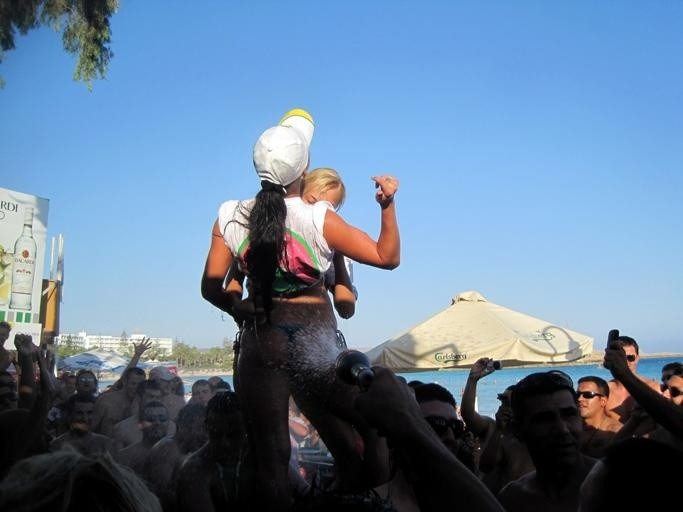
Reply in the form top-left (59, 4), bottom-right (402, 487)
top-left (223, 168), bottom-right (356, 512)
top-left (407, 335), bottom-right (683, 512)
top-left (0, 322), bottom-right (329, 512)
top-left (203, 108), bottom-right (401, 507)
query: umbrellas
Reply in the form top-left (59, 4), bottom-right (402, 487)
top-left (359, 290), bottom-right (597, 414)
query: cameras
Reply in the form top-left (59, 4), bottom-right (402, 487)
top-left (487, 360), bottom-right (502, 370)
top-left (464, 431), bottom-right (474, 440)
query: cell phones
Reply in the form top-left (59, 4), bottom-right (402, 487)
top-left (607, 329), bottom-right (619, 349)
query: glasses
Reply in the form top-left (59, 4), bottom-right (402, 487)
top-left (77, 375), bottom-right (95, 385)
top-left (0, 391), bottom-right (16, 403)
top-left (575, 389), bottom-right (606, 401)
top-left (421, 415), bottom-right (465, 443)
top-left (141, 415), bottom-right (169, 421)
top-left (623, 355), bottom-right (636, 363)
top-left (659, 383), bottom-right (682, 399)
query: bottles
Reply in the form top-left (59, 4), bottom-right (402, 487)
top-left (8, 207), bottom-right (35, 309)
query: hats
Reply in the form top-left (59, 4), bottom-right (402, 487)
top-left (251, 108), bottom-right (316, 189)
top-left (147, 365), bottom-right (177, 384)
